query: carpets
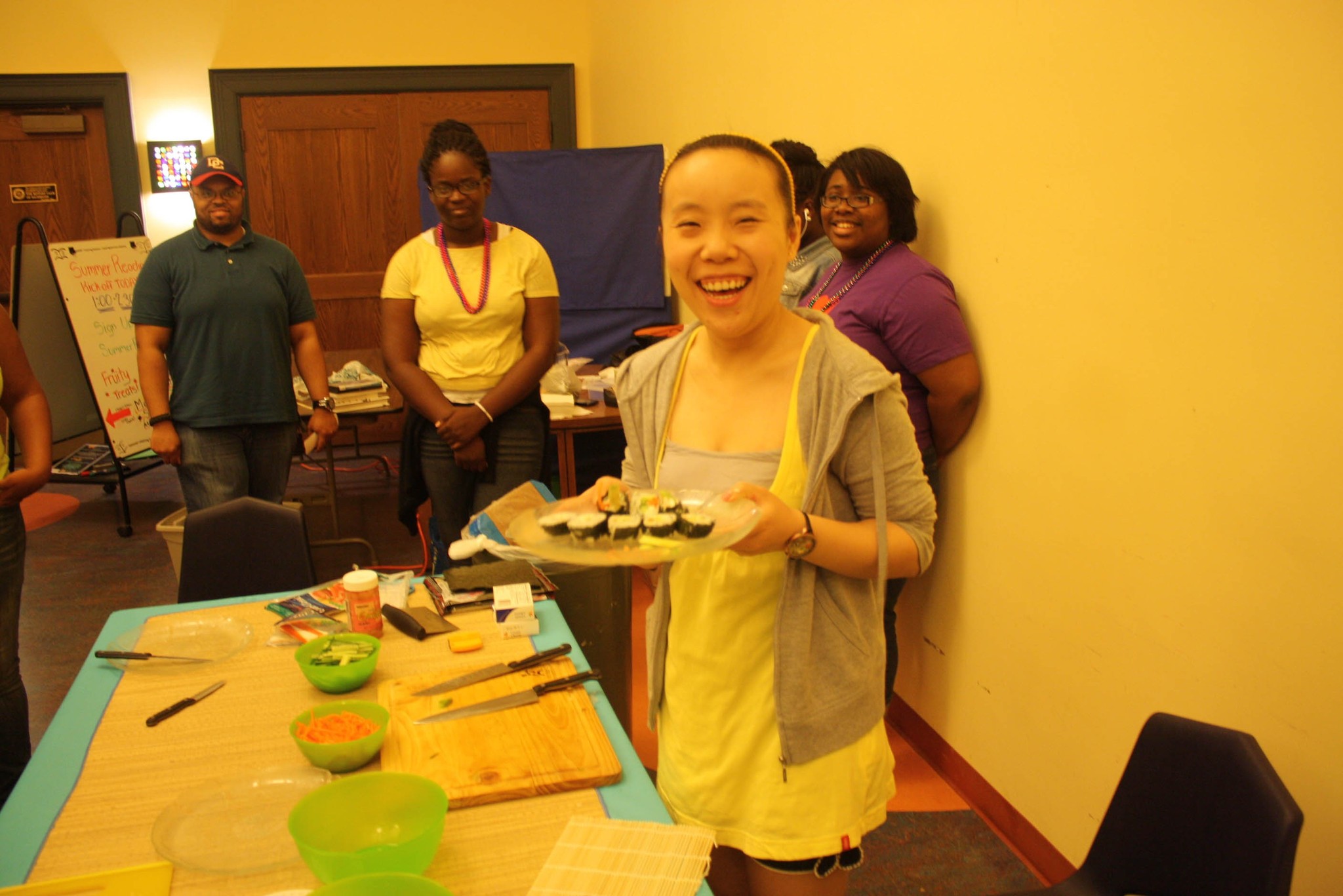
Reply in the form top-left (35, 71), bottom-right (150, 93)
top-left (847, 809), bottom-right (1046, 896)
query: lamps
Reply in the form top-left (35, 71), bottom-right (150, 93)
top-left (145, 140), bottom-right (204, 194)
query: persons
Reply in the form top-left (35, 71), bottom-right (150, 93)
top-left (129, 155), bottom-right (338, 604)
top-left (613, 134), bottom-right (938, 896)
top-left (380, 119), bottom-right (560, 578)
top-left (0, 303), bottom-right (52, 812)
top-left (765, 138), bottom-right (984, 717)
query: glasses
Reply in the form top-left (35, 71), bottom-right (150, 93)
top-left (191, 187), bottom-right (243, 200)
top-left (427, 177), bottom-right (486, 198)
top-left (820, 194), bottom-right (886, 208)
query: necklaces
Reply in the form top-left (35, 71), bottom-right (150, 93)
top-left (808, 239), bottom-right (894, 312)
top-left (437, 217), bottom-right (492, 315)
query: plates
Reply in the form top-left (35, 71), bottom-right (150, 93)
top-left (507, 489), bottom-right (759, 563)
top-left (152, 764), bottom-right (351, 874)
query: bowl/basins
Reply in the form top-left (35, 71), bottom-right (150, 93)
top-left (289, 698), bottom-right (388, 774)
top-left (294, 633), bottom-right (381, 693)
top-left (285, 771), bottom-right (448, 883)
top-left (304, 872), bottom-right (456, 895)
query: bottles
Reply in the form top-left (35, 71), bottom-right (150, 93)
top-left (341, 569), bottom-right (384, 639)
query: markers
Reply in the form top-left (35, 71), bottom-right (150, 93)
top-left (90, 466), bottom-right (130, 476)
top-left (81, 467), bottom-right (114, 476)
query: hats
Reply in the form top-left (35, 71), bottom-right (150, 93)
top-left (189, 157), bottom-right (245, 189)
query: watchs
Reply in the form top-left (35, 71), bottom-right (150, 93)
top-left (784, 512), bottom-right (817, 560)
top-left (312, 396), bottom-right (335, 412)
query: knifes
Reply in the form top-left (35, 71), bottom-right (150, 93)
top-left (94, 650), bottom-right (214, 663)
top-left (407, 667), bottom-right (603, 726)
top-left (409, 643), bottom-right (572, 696)
top-left (145, 679), bottom-right (226, 727)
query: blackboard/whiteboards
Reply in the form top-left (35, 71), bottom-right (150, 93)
top-left (4, 235), bottom-right (173, 483)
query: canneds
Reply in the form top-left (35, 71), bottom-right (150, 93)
top-left (342, 569), bottom-right (384, 639)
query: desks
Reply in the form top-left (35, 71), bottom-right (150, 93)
top-left (297, 384), bottom-right (405, 567)
top-left (548, 364), bottom-right (624, 498)
top-left (0, 558), bottom-right (716, 896)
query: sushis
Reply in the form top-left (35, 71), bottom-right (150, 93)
top-left (538, 484), bottom-right (716, 538)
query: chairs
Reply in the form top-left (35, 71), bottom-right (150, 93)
top-left (177, 497), bottom-right (314, 604)
top-left (1018, 710), bottom-right (1304, 896)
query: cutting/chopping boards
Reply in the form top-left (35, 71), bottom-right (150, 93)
top-left (375, 650), bottom-right (622, 809)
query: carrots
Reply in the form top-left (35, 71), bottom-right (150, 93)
top-left (295, 710), bottom-right (380, 744)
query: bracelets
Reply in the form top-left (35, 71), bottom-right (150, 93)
top-left (149, 413), bottom-right (171, 426)
top-left (474, 401), bottom-right (493, 422)
